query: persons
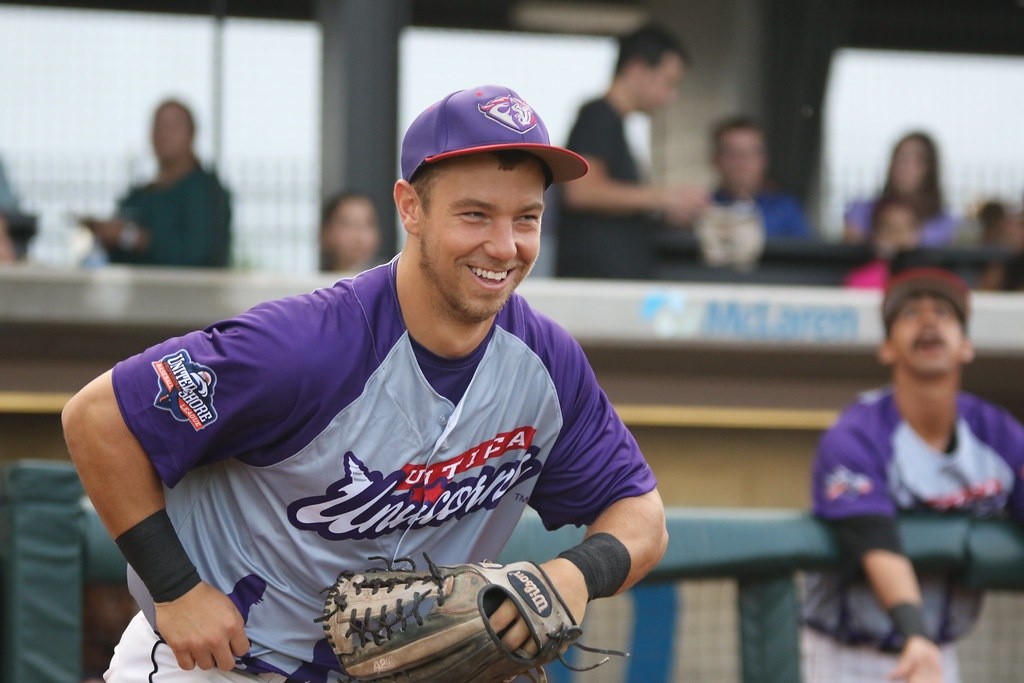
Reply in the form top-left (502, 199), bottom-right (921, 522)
top-left (798, 269), bottom-right (1024, 683)
top-left (61, 82), bottom-right (670, 683)
top-left (1, 27), bottom-right (1024, 290)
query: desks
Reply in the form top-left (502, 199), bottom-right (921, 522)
top-left (648, 234), bottom-right (1013, 289)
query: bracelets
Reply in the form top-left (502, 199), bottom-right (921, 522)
top-left (122, 226), bottom-right (140, 249)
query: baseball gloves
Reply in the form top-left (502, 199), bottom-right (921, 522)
top-left (313, 550), bottom-right (630, 683)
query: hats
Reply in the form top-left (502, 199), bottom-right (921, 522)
top-left (883, 268), bottom-right (968, 324)
top-left (403, 86), bottom-right (590, 186)
